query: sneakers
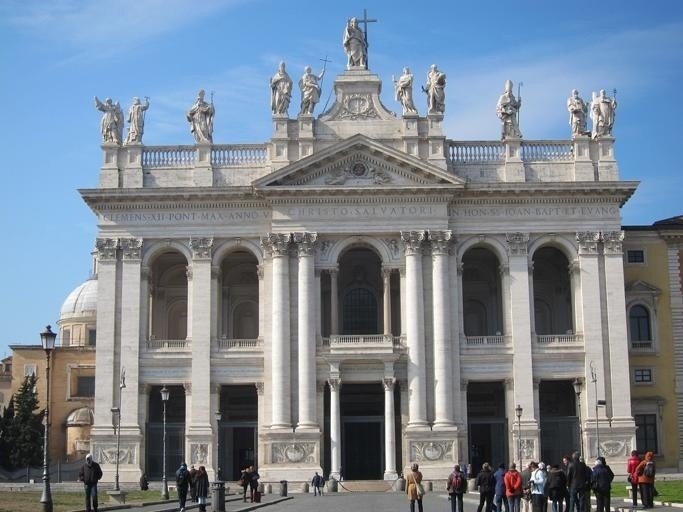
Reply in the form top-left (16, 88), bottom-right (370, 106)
top-left (180, 508), bottom-right (185, 512)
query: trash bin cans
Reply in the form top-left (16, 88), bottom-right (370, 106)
top-left (279, 481), bottom-right (287, 497)
top-left (211, 481), bottom-right (225, 512)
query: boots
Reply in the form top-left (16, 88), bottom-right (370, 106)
top-left (198, 504), bottom-right (206, 512)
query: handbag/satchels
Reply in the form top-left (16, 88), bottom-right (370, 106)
top-left (628, 475), bottom-right (632, 482)
top-left (417, 483), bottom-right (425, 496)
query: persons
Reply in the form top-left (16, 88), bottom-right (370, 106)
top-left (79, 454), bottom-right (103, 512)
top-left (495, 79), bottom-right (523, 139)
top-left (343, 15), bottom-right (367, 68)
top-left (311, 472), bottom-right (325, 496)
top-left (425, 64), bottom-right (447, 112)
top-left (447, 452), bottom-right (614, 512)
top-left (626, 450), bottom-right (656, 508)
top-left (139, 472), bottom-right (150, 490)
top-left (405, 463), bottom-right (423, 512)
top-left (240, 465), bottom-right (259, 503)
top-left (124, 96), bottom-right (149, 144)
top-left (269, 61), bottom-right (293, 115)
top-left (184, 89), bottom-right (215, 143)
top-left (590, 87), bottom-right (617, 138)
top-left (175, 462), bottom-right (209, 512)
top-left (567, 89), bottom-right (589, 133)
top-left (93, 95), bottom-right (124, 143)
top-left (394, 65), bottom-right (419, 114)
top-left (297, 66), bottom-right (325, 115)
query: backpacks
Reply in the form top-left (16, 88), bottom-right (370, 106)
top-left (643, 461), bottom-right (655, 478)
top-left (176, 472), bottom-right (186, 486)
top-left (451, 471), bottom-right (463, 488)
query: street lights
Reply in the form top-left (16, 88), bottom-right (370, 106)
top-left (215, 410), bottom-right (223, 480)
top-left (515, 405), bottom-right (523, 472)
top-left (571, 376), bottom-right (583, 462)
top-left (159, 383), bottom-right (170, 501)
top-left (40, 323), bottom-right (58, 512)
top-left (109, 407), bottom-right (120, 490)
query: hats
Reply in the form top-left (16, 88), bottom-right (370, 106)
top-left (573, 451), bottom-right (579, 460)
